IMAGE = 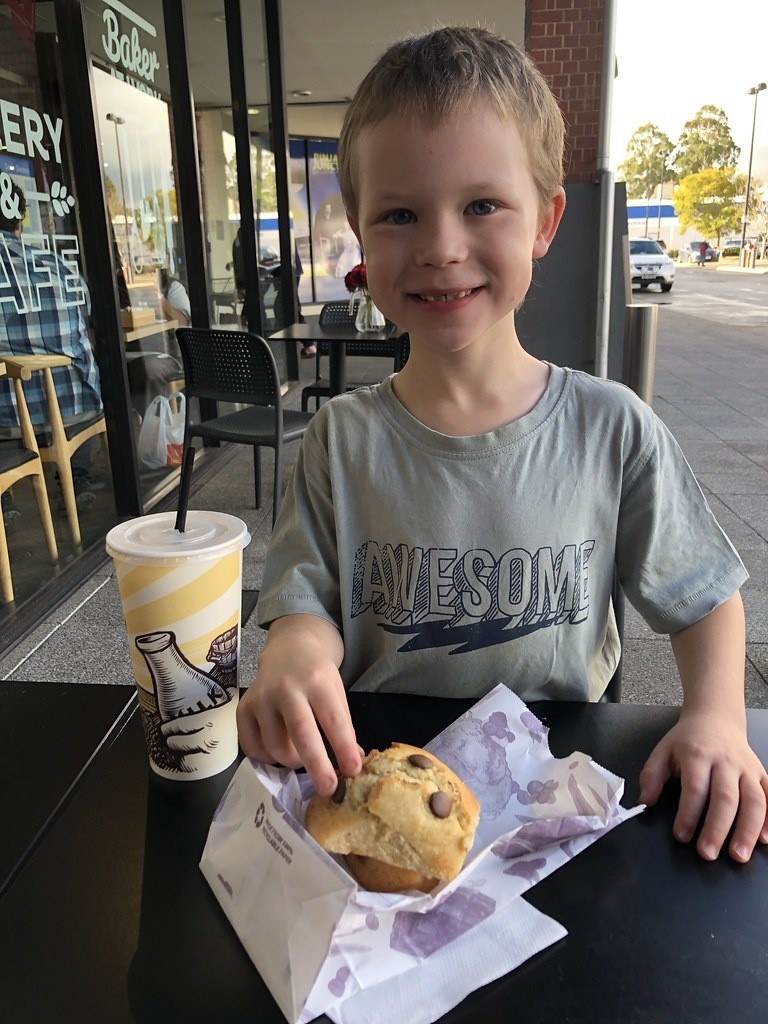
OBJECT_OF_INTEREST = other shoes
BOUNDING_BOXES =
[57,479,96,517]
[0,491,21,525]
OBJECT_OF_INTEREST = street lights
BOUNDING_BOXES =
[740,81,768,252]
[105,111,135,283]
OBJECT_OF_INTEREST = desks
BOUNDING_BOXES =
[87,319,180,342]
[0,679,139,887]
[269,323,395,398]
[0,690,768,1024]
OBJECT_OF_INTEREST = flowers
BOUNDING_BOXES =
[345,263,374,328]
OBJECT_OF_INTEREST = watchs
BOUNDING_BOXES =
[151,292,165,300]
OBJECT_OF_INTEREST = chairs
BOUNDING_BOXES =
[302,299,410,413]
[0,352,114,605]
[175,326,317,527]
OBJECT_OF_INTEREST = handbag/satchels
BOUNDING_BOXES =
[139,393,185,468]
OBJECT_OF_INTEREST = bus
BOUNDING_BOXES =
[230,210,296,271]
[112,213,179,274]
[724,236,757,249]
[627,197,702,253]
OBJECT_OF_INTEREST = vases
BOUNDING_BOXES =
[348,286,386,333]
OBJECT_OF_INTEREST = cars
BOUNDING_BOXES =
[655,240,666,250]
[678,240,716,263]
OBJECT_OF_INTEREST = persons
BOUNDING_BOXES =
[234,23,768,861]
[697,239,709,267]
[316,202,364,279]
[118,230,193,419]
[266,234,318,359]
[0,178,109,526]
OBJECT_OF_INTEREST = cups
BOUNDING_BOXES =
[106,509,253,782]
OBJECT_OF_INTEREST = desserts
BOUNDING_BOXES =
[304,742,480,892]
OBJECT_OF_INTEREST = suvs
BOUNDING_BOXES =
[630,236,675,293]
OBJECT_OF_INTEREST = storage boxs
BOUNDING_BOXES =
[120,309,156,327]
